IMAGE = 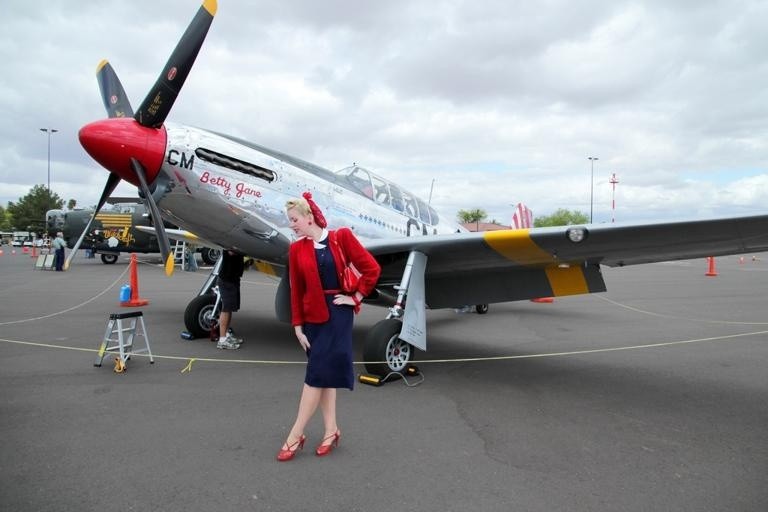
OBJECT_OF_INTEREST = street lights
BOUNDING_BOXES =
[588,156,599,224]
[39,128,58,199]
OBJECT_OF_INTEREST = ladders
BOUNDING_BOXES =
[94,311,154,370]
[173,226,186,271]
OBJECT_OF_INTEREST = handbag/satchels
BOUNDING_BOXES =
[334,228,363,293]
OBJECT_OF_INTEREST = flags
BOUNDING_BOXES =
[508,203,533,230]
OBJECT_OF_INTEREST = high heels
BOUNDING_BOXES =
[277,433,306,461]
[316,429,341,456]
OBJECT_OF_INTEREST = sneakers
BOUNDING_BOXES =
[217,335,244,350]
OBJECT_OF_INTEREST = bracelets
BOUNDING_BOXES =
[351,293,362,314]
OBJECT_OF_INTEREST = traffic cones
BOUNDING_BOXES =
[705,257,719,277]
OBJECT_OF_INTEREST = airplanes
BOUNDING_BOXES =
[63,0,768,381]
[41,195,255,271]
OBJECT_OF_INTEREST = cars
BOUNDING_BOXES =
[13,240,34,247]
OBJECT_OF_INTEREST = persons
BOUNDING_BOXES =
[216,249,245,351]
[20,236,26,247]
[274,195,383,462]
[52,232,68,271]
